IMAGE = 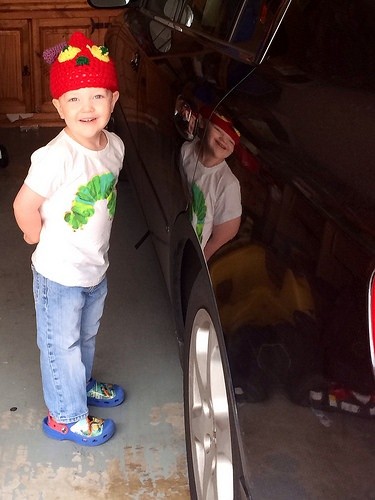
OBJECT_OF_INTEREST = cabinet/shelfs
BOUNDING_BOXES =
[0,0,125,128]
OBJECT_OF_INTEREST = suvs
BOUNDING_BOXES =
[89,0,375,500]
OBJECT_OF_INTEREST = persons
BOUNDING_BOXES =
[12,31,126,448]
[179,104,242,263]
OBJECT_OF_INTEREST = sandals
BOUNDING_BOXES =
[42,409,115,446]
[87,378,126,407]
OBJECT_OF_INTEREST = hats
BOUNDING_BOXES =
[200,104,241,146]
[49,31,120,99]
